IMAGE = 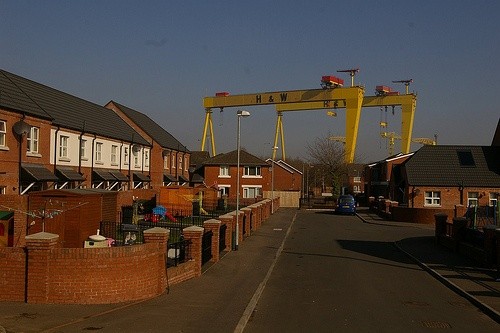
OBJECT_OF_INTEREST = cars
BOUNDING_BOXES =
[335,195,356,215]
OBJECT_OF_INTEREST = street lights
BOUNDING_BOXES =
[235,109,249,250]
